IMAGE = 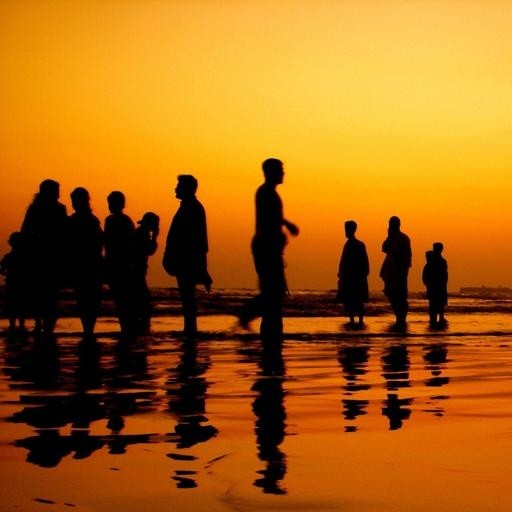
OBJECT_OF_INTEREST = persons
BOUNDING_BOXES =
[161,174,213,332]
[336,220,370,330]
[379,215,413,334]
[132,212,160,335]
[432,242,448,328]
[103,190,146,338]
[238,157,299,337]
[0,178,69,342]
[422,250,444,329]
[67,187,104,336]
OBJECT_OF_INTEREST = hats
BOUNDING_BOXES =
[137,212,159,224]
